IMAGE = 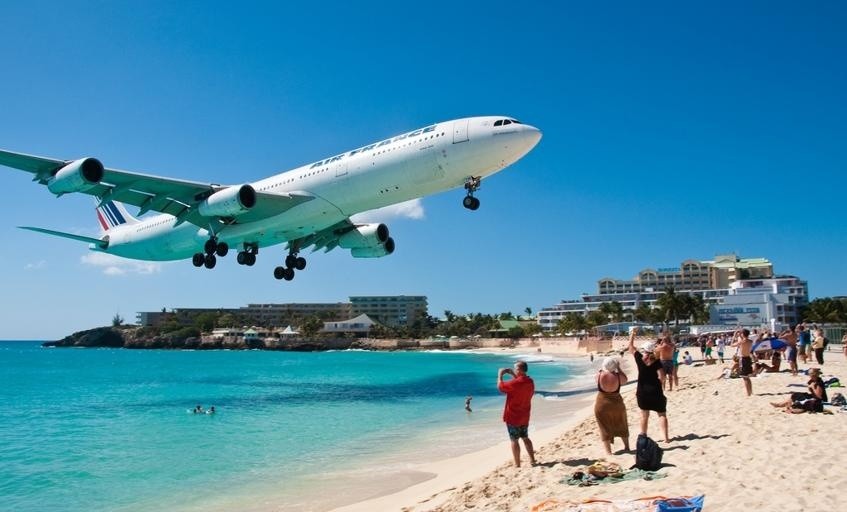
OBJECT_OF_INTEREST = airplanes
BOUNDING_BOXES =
[0,113,548,285]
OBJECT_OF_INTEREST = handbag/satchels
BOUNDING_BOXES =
[652,493,705,512]
[588,461,625,479]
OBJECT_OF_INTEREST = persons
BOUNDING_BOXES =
[653,321,846,397]
[197,406,205,413]
[593,355,630,457]
[627,322,672,445]
[193,409,204,414]
[768,367,827,414]
[464,396,473,413]
[495,361,537,469]
[206,406,215,414]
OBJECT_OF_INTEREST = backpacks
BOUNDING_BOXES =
[629,434,663,471]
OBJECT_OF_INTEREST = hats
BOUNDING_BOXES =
[640,341,656,353]
[602,357,619,374]
